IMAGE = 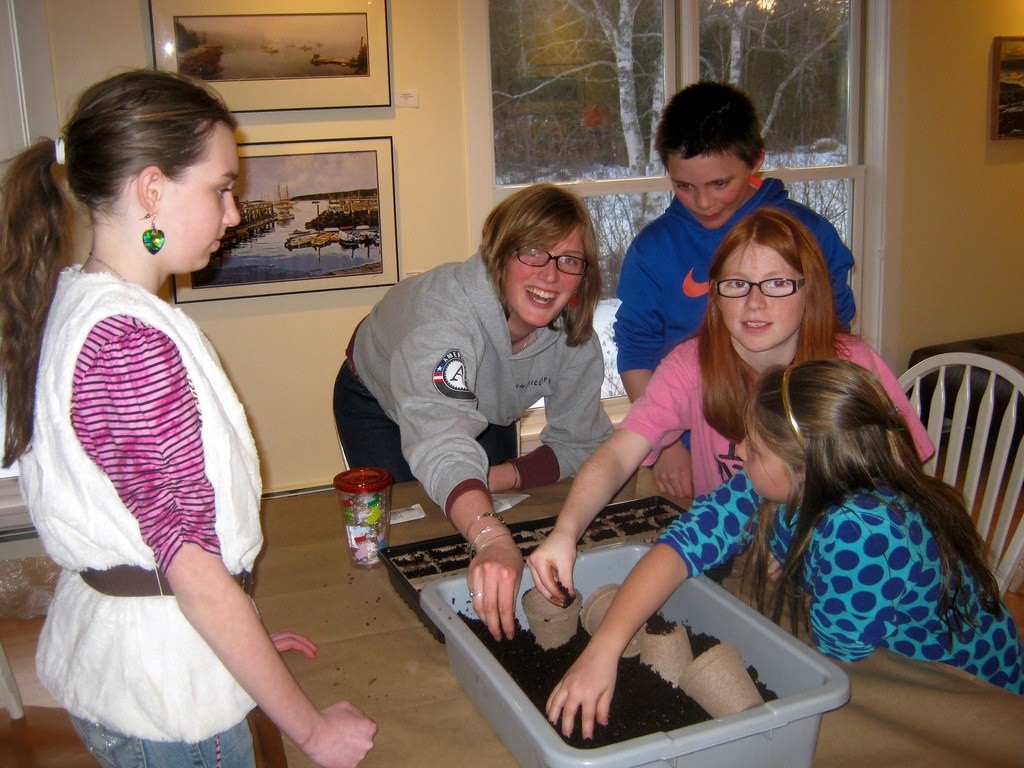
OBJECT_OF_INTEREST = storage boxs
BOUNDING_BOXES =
[378,495,734,644]
[419,540,854,768]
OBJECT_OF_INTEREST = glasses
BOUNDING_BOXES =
[515,246,588,275]
[714,278,805,298]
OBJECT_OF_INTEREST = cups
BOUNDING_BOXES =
[581,584,656,658]
[521,584,582,652]
[333,467,392,569]
[640,624,694,689]
[678,642,764,718]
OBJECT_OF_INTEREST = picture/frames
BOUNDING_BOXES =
[148,0,391,113]
[173,136,399,304]
[991,35,1024,140]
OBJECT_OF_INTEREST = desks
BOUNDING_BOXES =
[252,467,1024,768]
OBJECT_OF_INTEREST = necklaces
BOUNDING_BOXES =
[522,331,533,350]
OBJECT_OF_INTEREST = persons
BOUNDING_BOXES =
[333,183,615,641]
[612,80,856,499]
[544,357,1024,742]
[0,68,378,768]
[528,208,936,608]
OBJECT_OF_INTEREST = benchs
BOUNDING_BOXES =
[909,333,1024,445]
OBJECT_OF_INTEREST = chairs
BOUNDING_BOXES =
[898,352,1024,601]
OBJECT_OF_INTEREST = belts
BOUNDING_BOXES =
[79,564,252,597]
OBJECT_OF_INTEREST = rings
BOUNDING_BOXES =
[469,592,483,596]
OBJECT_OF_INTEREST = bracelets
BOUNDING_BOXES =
[466,513,511,560]
[506,461,517,489]
